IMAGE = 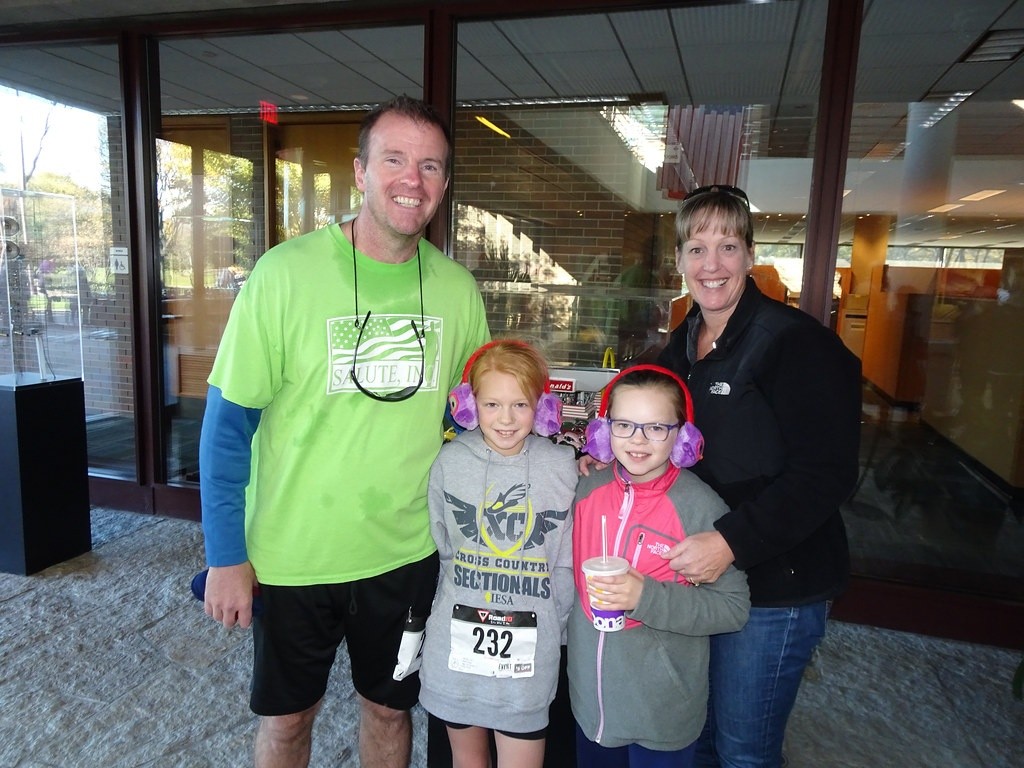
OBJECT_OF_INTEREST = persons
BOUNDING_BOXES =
[418,336,583,768]
[566,364,750,768]
[577,185,862,768]
[613,248,668,358]
[192,93,493,768]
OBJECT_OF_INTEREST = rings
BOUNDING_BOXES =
[688,577,700,587]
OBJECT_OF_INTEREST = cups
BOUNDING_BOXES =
[581,556,629,632]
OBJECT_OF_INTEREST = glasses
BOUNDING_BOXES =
[673,183,751,202]
[349,309,425,402]
[606,413,684,443]
